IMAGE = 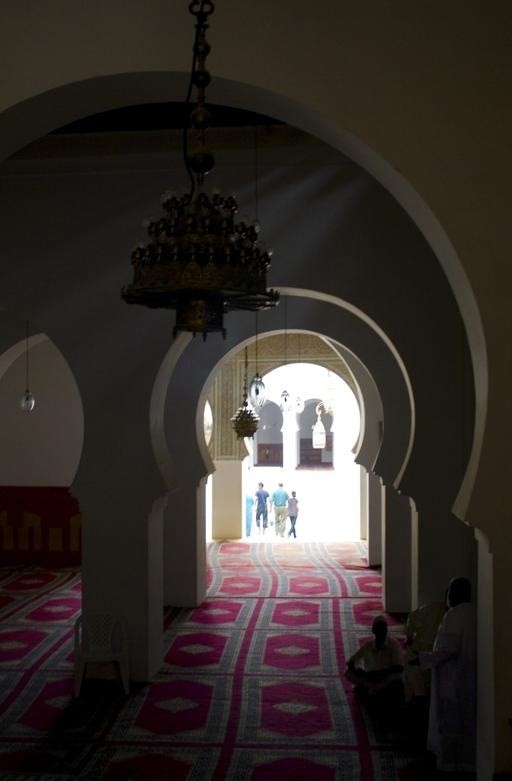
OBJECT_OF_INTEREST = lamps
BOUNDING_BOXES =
[247,312,269,409]
[311,401,331,450]
[21,321,37,415]
[117,0,282,347]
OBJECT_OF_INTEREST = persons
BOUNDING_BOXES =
[345,574,478,781]
[246,495,254,537]
[255,482,269,534]
[288,491,298,538]
[269,482,289,538]
